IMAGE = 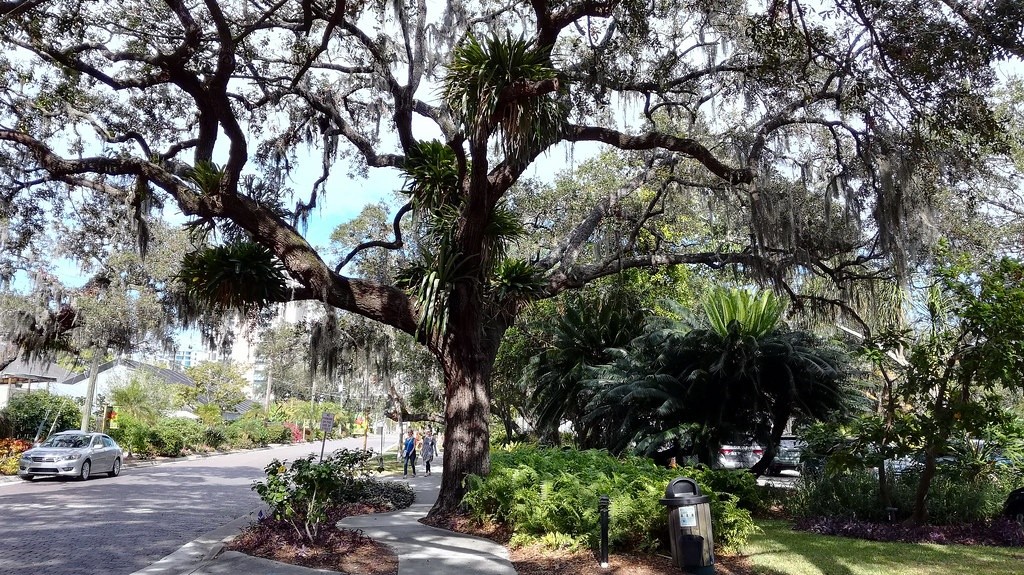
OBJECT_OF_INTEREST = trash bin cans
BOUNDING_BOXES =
[655,478,715,574]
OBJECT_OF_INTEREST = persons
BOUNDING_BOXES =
[403,428,438,479]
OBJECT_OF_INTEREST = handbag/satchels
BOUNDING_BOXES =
[401,450,408,458]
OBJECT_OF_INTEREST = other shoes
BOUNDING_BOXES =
[427,471,432,475]
[403,474,408,479]
[424,472,428,477]
[411,473,415,478]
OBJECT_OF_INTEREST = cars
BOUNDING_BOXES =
[718,432,807,479]
[16,432,123,482]
